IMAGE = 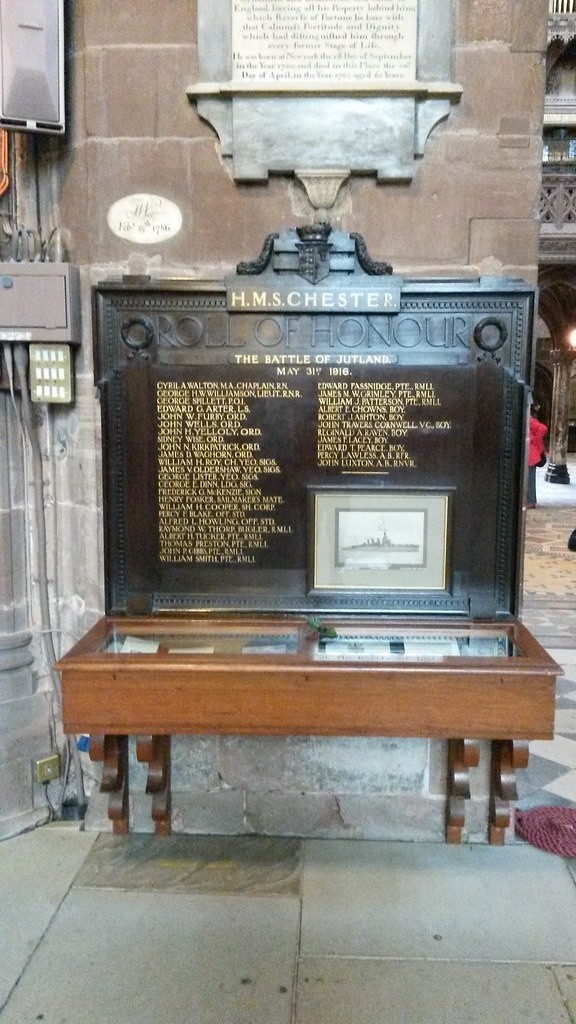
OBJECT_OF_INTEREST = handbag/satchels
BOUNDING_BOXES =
[536,451,546,467]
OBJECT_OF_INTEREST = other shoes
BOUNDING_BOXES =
[526,503,534,509]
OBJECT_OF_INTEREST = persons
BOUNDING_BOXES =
[525,404,548,508]
